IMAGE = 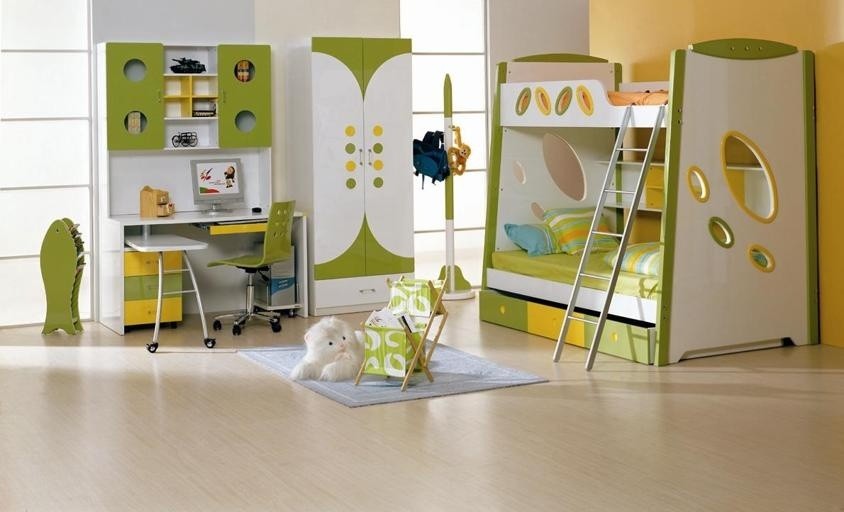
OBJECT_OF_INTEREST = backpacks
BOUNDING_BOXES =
[414,131,450,183]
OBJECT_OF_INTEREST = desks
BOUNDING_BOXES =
[125,233,217,354]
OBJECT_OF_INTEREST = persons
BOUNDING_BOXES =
[224,165,235,179]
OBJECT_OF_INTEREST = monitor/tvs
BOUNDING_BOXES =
[189,158,245,215]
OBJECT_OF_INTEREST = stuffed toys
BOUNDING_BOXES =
[446,126,472,175]
[290,316,368,383]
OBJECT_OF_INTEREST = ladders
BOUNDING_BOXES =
[551,105,667,372]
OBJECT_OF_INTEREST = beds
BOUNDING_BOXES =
[478,37,821,367]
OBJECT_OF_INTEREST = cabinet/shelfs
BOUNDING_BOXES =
[354,274,450,392]
[41,218,87,336]
[288,36,416,318]
[97,41,309,337]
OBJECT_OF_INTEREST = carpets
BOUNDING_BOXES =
[236,336,550,409]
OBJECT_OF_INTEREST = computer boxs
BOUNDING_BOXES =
[254,243,295,307]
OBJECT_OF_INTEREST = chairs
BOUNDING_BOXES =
[205,199,297,336]
